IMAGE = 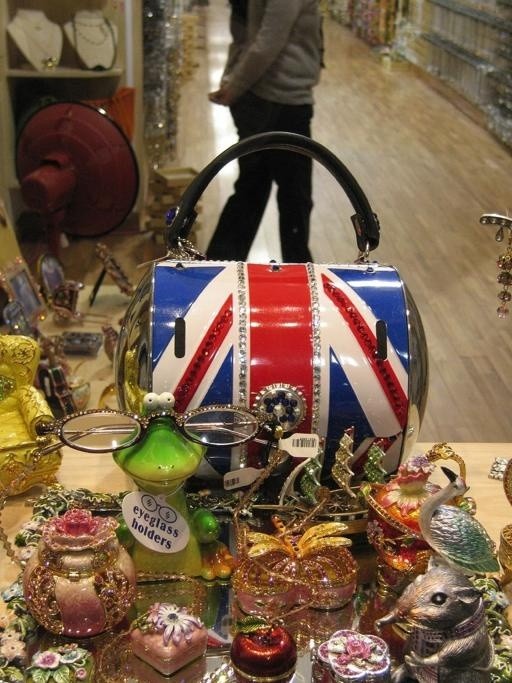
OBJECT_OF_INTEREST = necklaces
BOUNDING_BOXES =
[73,13,118,69]
[16,12,60,74]
[18,14,49,32]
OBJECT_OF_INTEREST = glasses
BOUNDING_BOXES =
[32,403,277,463]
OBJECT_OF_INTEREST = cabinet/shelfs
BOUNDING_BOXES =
[382,0,512,149]
[1,0,149,292]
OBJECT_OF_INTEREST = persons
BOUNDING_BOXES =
[205,0,327,263]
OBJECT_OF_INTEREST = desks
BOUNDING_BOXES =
[1,283,512,683]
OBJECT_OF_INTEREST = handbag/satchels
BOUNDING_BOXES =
[114,130,429,474]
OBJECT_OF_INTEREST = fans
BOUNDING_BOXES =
[14,100,140,265]
